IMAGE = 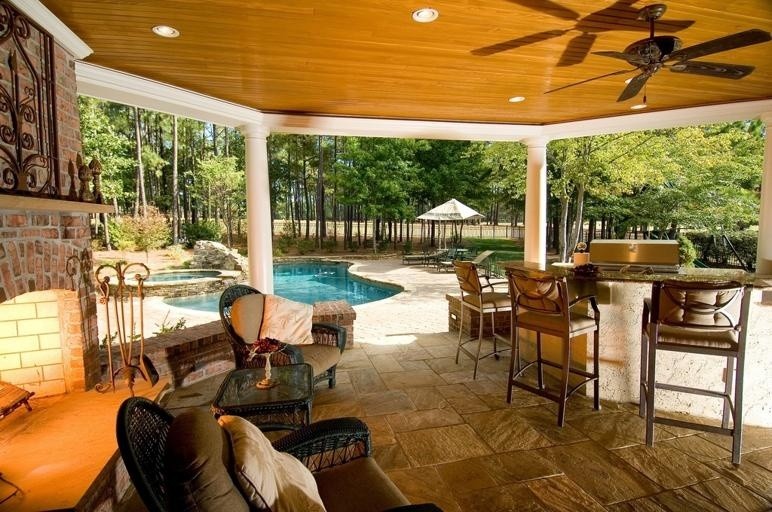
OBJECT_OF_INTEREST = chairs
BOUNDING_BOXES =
[405,245,498,278]
[501,262,606,426]
[115,394,440,511]
[638,278,753,468]
[452,257,514,383]
[217,281,345,395]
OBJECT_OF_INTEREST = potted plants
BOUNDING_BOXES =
[572,240,590,266]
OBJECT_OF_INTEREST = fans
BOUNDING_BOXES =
[543,2,770,101]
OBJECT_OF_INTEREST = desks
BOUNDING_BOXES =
[543,255,771,431]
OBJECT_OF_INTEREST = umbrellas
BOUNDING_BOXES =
[416,198,486,251]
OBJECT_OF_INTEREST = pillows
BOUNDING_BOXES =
[256,295,316,349]
[162,406,248,512]
[229,291,263,344]
[217,412,327,512]
